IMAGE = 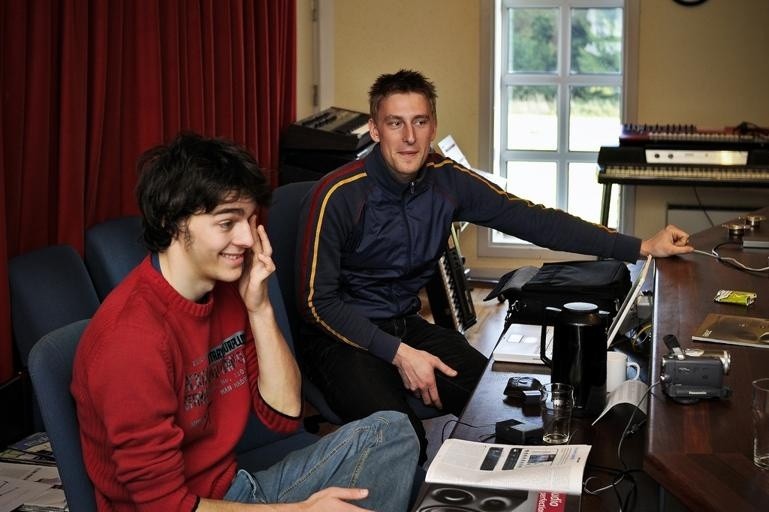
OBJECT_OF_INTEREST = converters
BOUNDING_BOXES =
[495,417,543,446]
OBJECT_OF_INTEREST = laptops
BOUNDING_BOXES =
[493,255,652,365]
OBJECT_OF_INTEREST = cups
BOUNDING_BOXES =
[539,382,575,444]
[751,378,769,472]
[607,352,640,394]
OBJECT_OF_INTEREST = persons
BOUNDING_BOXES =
[71,131,420,512]
[293,68,694,420]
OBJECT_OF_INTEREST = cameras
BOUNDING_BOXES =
[660,335,734,406]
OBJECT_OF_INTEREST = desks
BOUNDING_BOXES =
[411,216,769,512]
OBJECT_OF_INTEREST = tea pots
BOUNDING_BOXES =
[539,300,611,411]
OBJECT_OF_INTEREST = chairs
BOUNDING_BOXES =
[268,181,448,435]
[8,245,100,366]
[84,216,150,305]
[28,318,96,512]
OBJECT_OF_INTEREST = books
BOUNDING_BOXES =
[413,437,597,512]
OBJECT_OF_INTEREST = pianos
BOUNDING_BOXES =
[425,222,477,335]
[280,106,377,182]
[598,147,769,187]
[619,122,767,150]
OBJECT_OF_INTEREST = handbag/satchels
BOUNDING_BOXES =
[483,259,630,313]
